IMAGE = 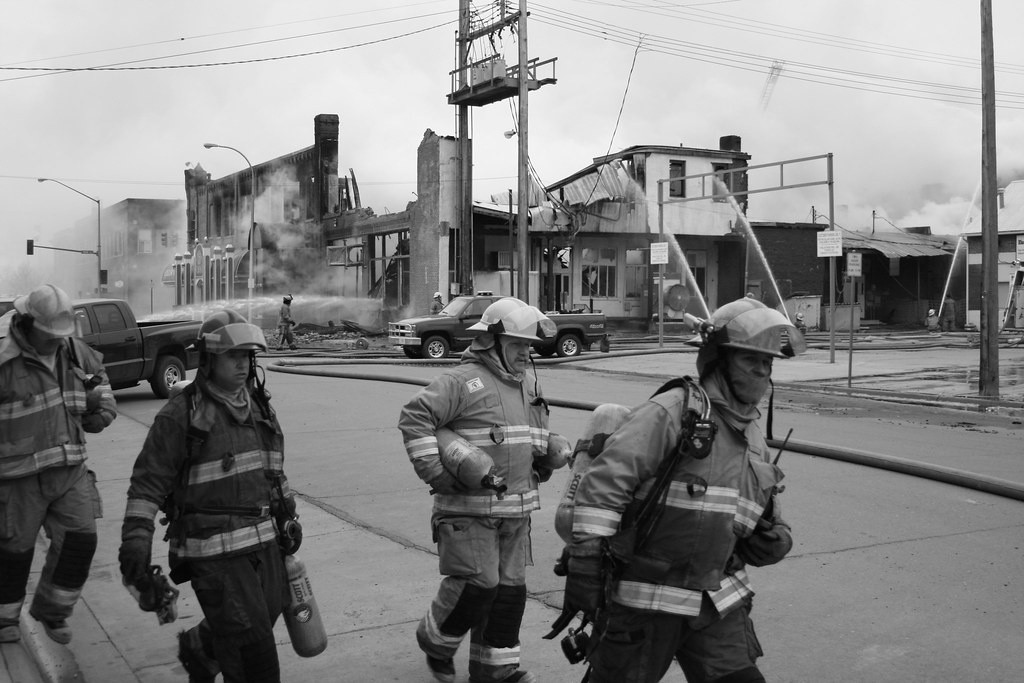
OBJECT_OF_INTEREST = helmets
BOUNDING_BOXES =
[183,310,264,353]
[13,284,76,336]
[465,295,543,341]
[683,297,790,359]
[283,293,293,300]
[433,291,442,300]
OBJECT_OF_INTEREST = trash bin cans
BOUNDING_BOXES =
[775,292,823,332]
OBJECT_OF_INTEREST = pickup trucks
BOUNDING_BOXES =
[388,290,611,360]
[68,298,202,399]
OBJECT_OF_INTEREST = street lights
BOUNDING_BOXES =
[204,142,255,323]
[35,178,104,299]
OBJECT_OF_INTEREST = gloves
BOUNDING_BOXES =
[532,455,553,482]
[119,541,152,580]
[291,321,296,326]
[563,556,601,615]
[274,496,302,546]
[81,414,108,433]
[748,533,793,567]
[429,471,466,495]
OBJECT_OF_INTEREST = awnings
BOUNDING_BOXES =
[247,221,303,249]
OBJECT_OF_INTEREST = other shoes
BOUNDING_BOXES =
[178,632,216,683]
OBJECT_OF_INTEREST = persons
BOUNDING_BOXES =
[567,298,807,683]
[925,309,941,331]
[794,312,806,334]
[942,291,958,332]
[277,294,300,350]
[430,292,444,314]
[118,309,303,683]
[397,298,572,683]
[0,283,119,644]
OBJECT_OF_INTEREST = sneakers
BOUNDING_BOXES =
[0,620,20,643]
[426,654,456,683]
[469,670,536,683]
[29,607,72,643]
